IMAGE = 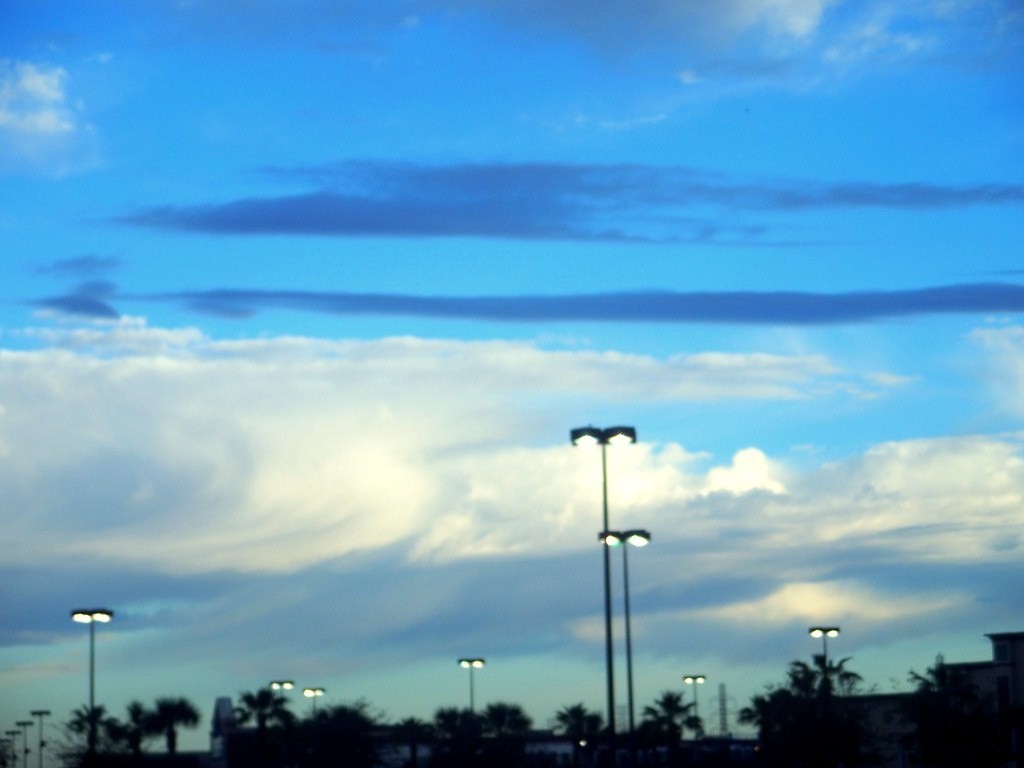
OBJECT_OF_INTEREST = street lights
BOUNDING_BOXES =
[70,607,115,710]
[268,680,295,710]
[596,529,654,768]
[567,425,640,768]
[31,709,52,768]
[806,624,841,680]
[303,687,327,722]
[16,720,35,768]
[5,730,22,768]
[681,674,707,743]
[456,656,486,712]
